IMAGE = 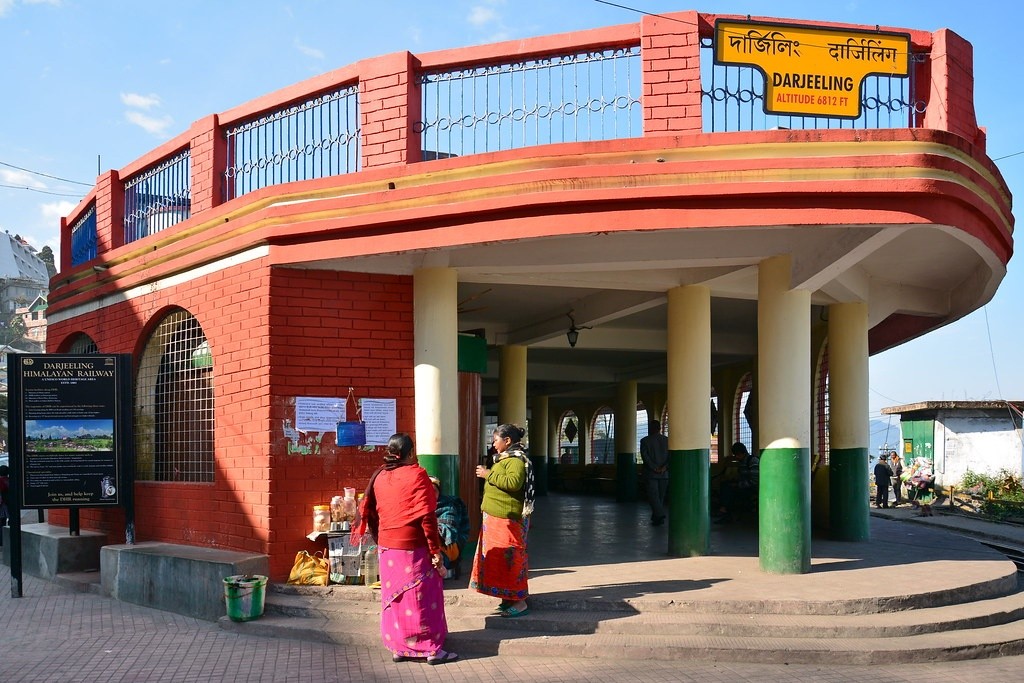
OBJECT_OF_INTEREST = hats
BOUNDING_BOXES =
[428,475,442,491]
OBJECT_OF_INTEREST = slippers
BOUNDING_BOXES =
[393,657,415,662]
[501,606,529,618]
[490,604,509,615]
[427,651,459,665]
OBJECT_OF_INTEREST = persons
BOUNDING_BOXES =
[561,448,574,465]
[874,455,894,509]
[640,420,671,526]
[0,465,9,546]
[711,442,760,524]
[349,432,459,665]
[428,475,470,570]
[466,424,535,619]
[889,451,907,507]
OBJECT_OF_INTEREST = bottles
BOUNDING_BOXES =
[365,546,378,585]
[313,486,365,533]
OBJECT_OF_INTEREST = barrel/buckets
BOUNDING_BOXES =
[223,575,268,622]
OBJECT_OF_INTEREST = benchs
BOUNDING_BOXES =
[588,477,615,493]
